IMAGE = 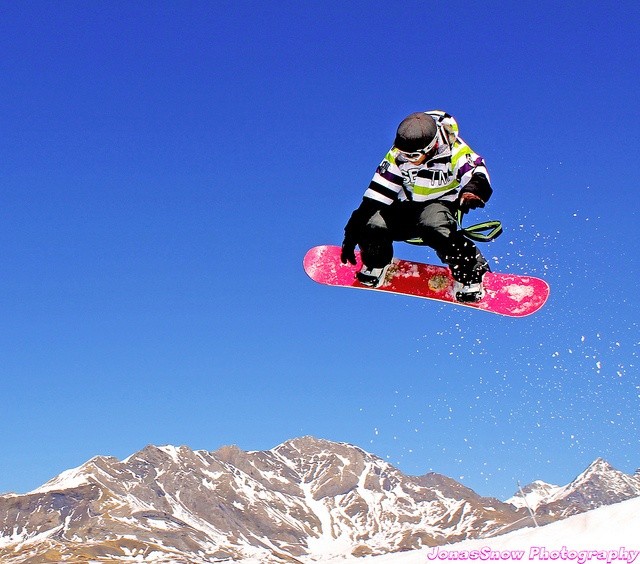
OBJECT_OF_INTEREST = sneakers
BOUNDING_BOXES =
[356,258,393,288]
[452,269,485,302]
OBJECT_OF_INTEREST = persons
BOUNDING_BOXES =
[340,111,494,303]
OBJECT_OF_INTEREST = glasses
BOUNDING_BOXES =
[390,146,424,162]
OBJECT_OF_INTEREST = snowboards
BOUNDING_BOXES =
[303,244,550,318]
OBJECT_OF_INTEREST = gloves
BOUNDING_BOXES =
[457,192,486,213]
[341,229,360,265]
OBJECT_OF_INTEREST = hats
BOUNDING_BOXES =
[395,113,438,153]
